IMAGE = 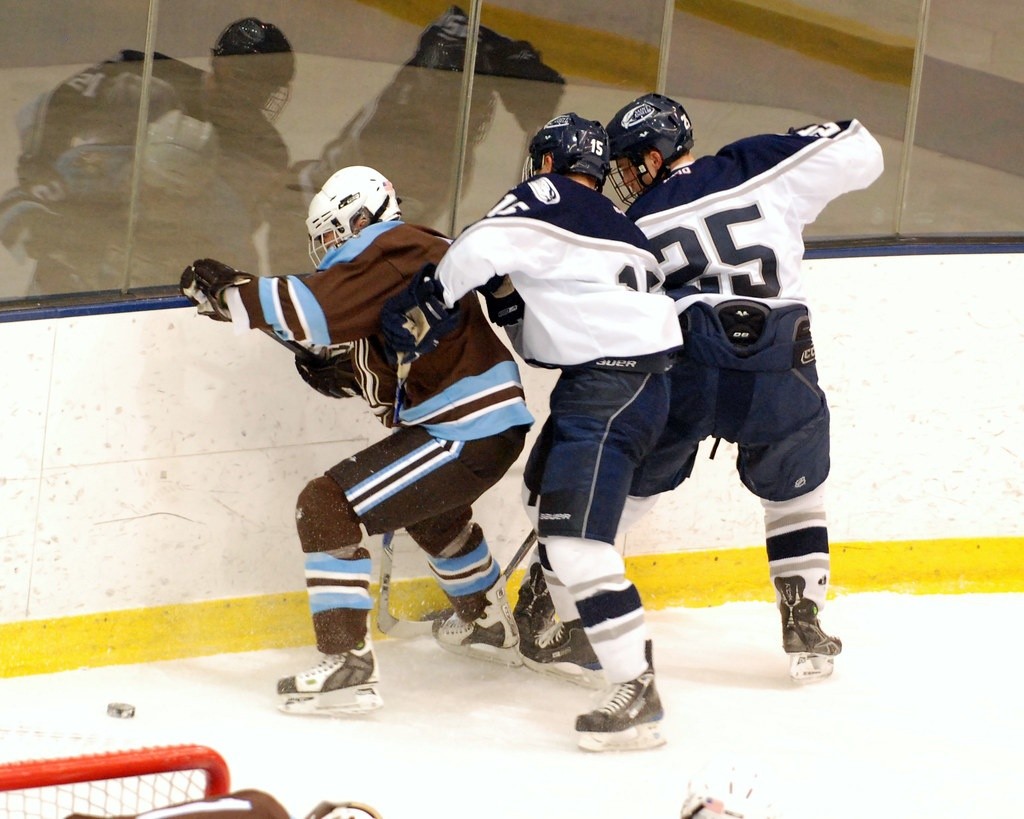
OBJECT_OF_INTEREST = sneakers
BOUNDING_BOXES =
[513,563,556,641]
[518,620,606,685]
[432,577,525,666]
[573,638,668,752]
[776,574,839,686]
[274,638,380,715]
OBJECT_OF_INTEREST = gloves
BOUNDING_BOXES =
[180,257,253,322]
[379,262,459,366]
[294,341,361,398]
[475,273,524,327]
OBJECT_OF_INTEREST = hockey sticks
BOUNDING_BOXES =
[432,529,536,639]
[255,319,320,362]
[376,352,446,637]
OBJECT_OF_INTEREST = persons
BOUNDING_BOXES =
[378,112,684,751]
[178,166,535,716]
[513,93,884,683]
[0,5,566,292]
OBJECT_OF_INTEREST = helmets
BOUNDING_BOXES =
[211,16,295,128]
[143,110,216,199]
[305,164,400,272]
[519,111,610,191]
[604,93,694,206]
[422,38,492,112]
